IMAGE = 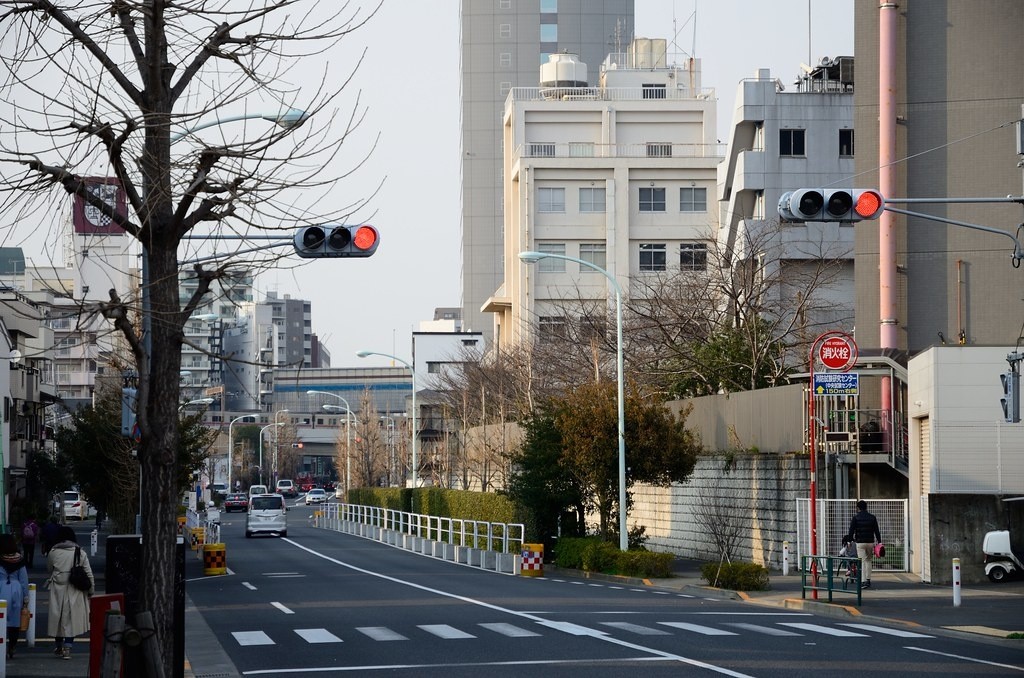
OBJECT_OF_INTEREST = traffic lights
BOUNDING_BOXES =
[999,370,1021,423]
[789,188,885,220]
[292,444,298,448]
[295,223,379,255]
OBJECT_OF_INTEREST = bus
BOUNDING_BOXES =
[225,493,249,513]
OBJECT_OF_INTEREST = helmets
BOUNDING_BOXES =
[875,544,885,558]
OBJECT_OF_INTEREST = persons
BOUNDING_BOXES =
[840,501,881,589]
[45,516,58,540]
[0,534,29,658]
[21,513,39,569]
[96,508,103,530]
[45,527,95,659]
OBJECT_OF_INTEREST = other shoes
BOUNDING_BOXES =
[62,648,72,659]
[846,570,850,575]
[7,646,17,654]
[54,647,63,656]
[861,579,871,589]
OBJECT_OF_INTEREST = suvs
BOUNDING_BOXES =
[276,479,299,498]
[206,482,227,500]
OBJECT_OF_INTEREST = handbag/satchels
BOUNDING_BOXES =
[19,602,30,632]
[69,547,92,592]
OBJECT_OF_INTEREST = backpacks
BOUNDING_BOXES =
[23,523,35,537]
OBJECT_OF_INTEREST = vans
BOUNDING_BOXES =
[248,484,268,500]
[245,493,291,538]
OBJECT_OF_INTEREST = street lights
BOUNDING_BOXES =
[275,409,290,486]
[354,350,417,490]
[381,416,395,469]
[142,105,313,380]
[229,413,260,493]
[517,250,629,555]
[306,390,351,490]
[322,404,356,441]
[259,422,286,485]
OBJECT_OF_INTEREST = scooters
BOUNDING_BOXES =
[983,529,1024,584]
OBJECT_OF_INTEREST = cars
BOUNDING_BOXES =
[305,488,328,505]
[55,491,89,521]
[302,482,334,493]
